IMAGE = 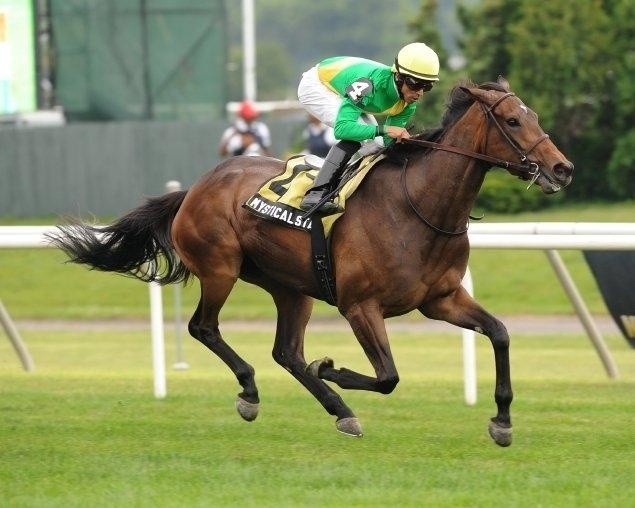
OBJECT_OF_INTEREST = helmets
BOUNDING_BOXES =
[389,41,442,82]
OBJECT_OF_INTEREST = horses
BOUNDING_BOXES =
[41,74,576,449]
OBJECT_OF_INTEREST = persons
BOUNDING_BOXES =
[301,112,342,159]
[219,100,271,159]
[298,42,440,213]
[237,129,266,156]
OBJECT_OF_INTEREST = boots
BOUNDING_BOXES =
[299,144,354,215]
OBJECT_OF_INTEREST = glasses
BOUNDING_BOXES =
[401,74,434,92]
